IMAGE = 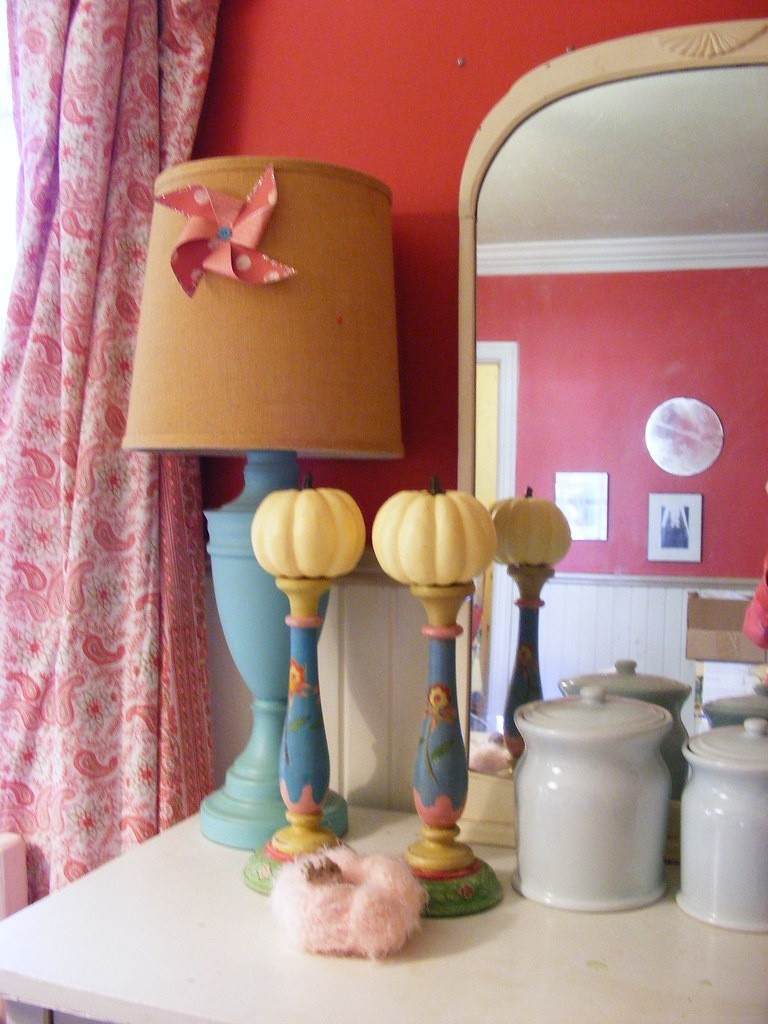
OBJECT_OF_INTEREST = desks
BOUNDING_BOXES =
[0,806,768,1024]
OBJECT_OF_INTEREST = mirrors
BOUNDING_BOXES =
[644,396,723,477]
[452,17,768,851]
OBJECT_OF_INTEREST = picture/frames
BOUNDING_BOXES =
[647,492,702,563]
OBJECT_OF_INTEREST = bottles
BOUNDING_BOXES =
[672,718,768,935]
[701,682,768,730]
[513,684,675,912]
[556,659,696,801]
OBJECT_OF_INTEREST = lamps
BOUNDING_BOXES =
[124,153,408,859]
